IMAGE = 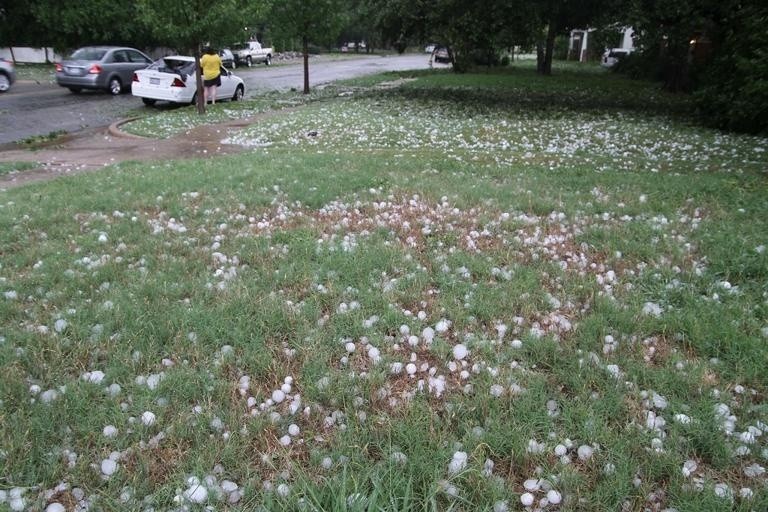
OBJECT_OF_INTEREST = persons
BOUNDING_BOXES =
[199,47,222,105]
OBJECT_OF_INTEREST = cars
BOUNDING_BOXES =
[217,47,237,69]
[435,47,452,65]
[131,55,246,106]
[0,57,17,92]
[56,45,155,95]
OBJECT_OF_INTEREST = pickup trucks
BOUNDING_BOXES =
[231,41,275,67]
